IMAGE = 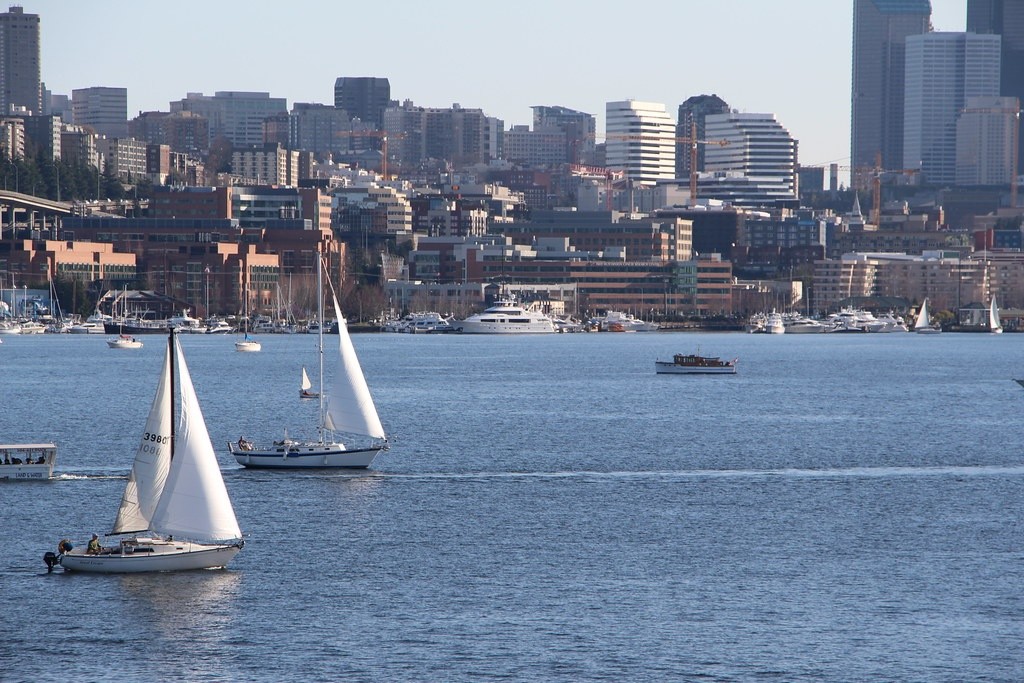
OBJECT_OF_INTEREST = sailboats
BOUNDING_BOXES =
[225,253,390,471]
[44,325,247,576]
[234,282,262,352]
[987,293,1006,335]
[298,366,320,399]
[106,296,144,349]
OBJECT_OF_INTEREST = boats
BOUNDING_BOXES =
[0,444,57,482]
[553,308,662,336]
[742,262,945,335]
[447,246,558,335]
[0,274,332,350]
[652,351,739,375]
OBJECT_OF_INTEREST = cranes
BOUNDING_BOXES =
[333,128,406,181]
[570,163,627,210]
[587,114,732,207]
[960,96,1024,210]
[792,148,920,231]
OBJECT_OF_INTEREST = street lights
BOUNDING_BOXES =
[88,163,98,201]
[44,164,60,203]
[10,162,19,193]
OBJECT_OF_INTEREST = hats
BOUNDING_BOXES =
[93,534,98,537]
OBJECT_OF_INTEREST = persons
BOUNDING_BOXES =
[238,436,249,451]
[87,533,106,555]
[5,457,44,465]
[303,390,308,395]
